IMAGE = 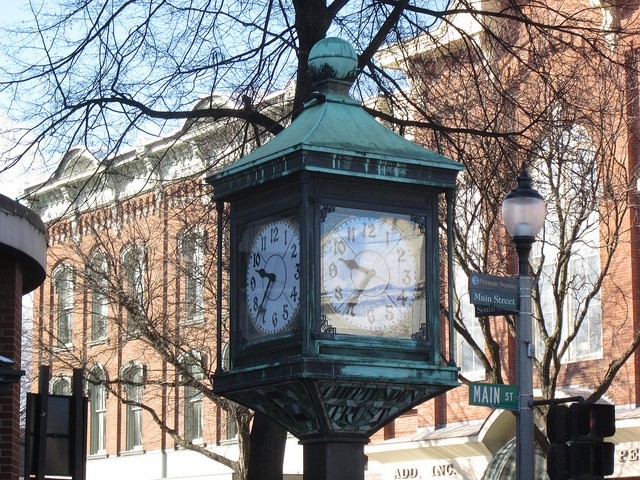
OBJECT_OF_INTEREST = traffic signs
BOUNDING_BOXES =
[468,272,519,296]
[468,381,519,410]
[474,305,519,317]
[470,288,520,313]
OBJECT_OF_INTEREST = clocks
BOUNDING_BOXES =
[314,196,433,349]
[231,197,304,353]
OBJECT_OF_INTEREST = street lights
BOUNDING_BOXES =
[502,159,546,480]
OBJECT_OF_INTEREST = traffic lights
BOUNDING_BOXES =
[570,401,616,479]
